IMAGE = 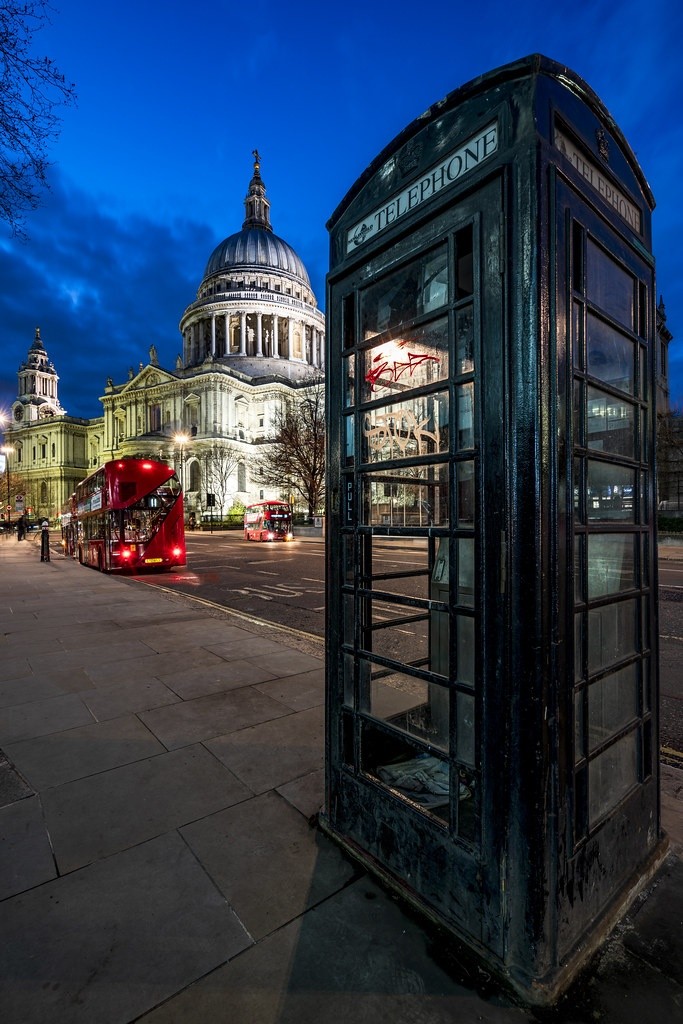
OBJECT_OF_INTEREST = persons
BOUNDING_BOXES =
[283,523,288,531]
[65,515,84,560]
[41,517,48,526]
[27,519,32,532]
[189,517,196,531]
[142,529,150,541]
[17,517,26,541]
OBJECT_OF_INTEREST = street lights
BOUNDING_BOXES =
[174,434,187,488]
[0,445,15,524]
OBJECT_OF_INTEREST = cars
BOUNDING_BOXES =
[0,522,40,533]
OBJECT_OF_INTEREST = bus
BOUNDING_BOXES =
[61,453,188,574]
[243,500,294,542]
